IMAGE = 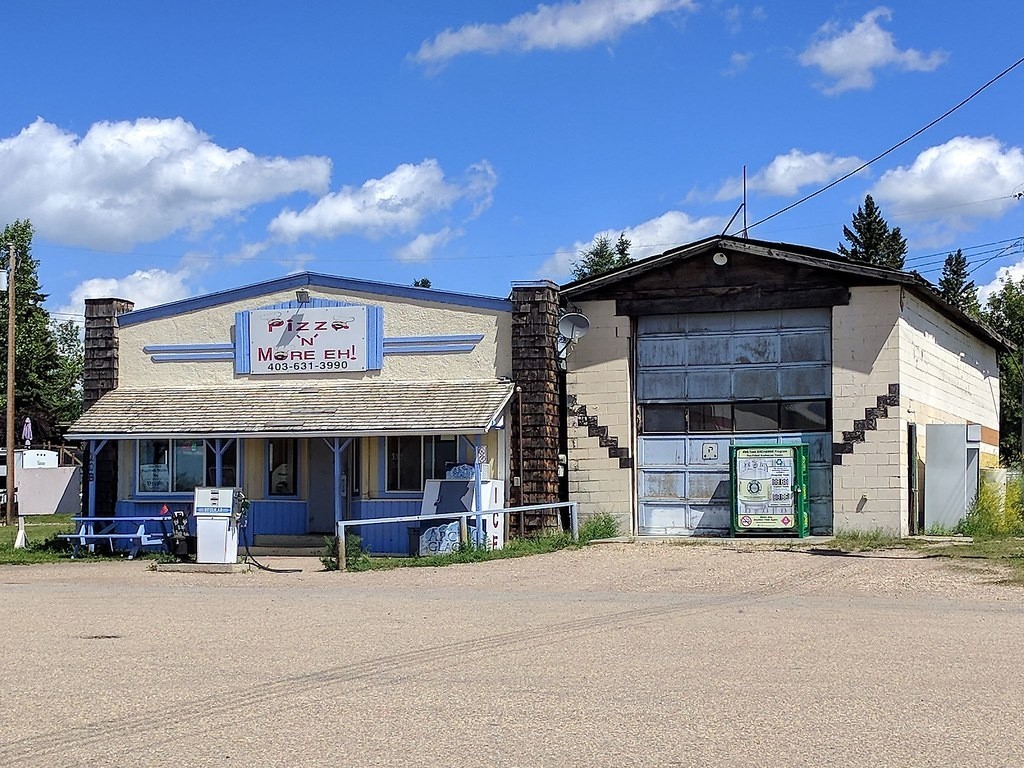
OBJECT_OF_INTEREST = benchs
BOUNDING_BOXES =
[56,534,152,539]
[151,533,173,537]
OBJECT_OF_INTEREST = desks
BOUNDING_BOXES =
[69,517,171,560]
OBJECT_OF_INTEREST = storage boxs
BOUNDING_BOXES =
[446,463,491,479]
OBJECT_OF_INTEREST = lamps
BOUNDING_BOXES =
[712,249,729,266]
[296,288,310,304]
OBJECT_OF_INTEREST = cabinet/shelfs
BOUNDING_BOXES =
[728,443,811,539]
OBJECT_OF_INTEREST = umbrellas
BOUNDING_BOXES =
[22,417,32,448]
